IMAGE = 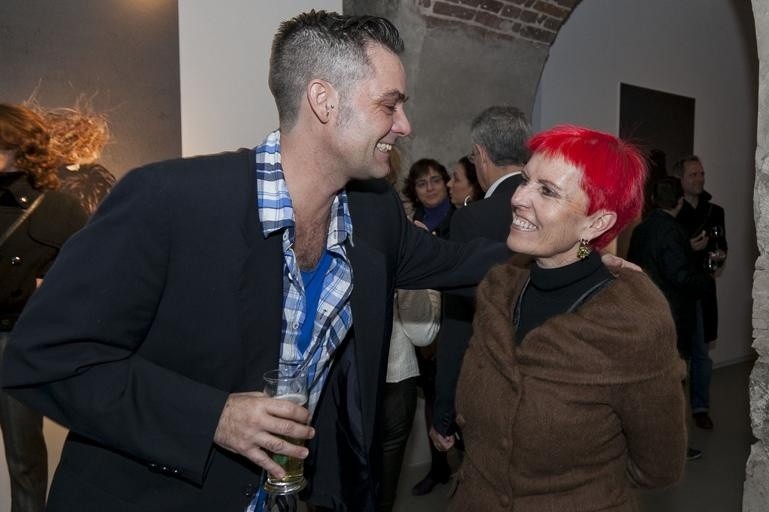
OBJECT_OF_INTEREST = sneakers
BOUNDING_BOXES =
[685,447,703,461]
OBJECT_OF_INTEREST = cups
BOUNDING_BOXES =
[263,368,307,496]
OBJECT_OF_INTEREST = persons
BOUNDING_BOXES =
[0,8,643,512]
[0,101,89,512]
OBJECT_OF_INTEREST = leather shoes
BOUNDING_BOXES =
[413,464,452,495]
[692,412,713,429]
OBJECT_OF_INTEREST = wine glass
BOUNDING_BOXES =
[703,258,718,281]
[711,225,724,256]
